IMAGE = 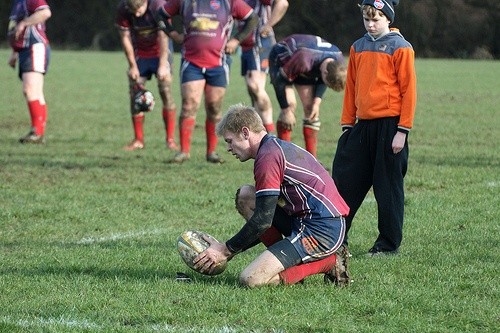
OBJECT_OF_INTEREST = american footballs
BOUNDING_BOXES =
[177,231,227,275]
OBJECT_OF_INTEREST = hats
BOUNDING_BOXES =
[133,82,155,111]
[361,0,399,23]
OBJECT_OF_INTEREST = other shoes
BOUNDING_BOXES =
[322,247,350,287]
[207,150,226,163]
[367,246,399,253]
[20,131,44,144]
[173,152,191,162]
[122,139,144,150]
[167,140,178,151]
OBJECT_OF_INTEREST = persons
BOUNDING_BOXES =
[268,34,348,159]
[332,0,417,253]
[235,0,290,134]
[114,0,179,151]
[192,102,350,288]
[8,0,51,144]
[154,0,259,164]
